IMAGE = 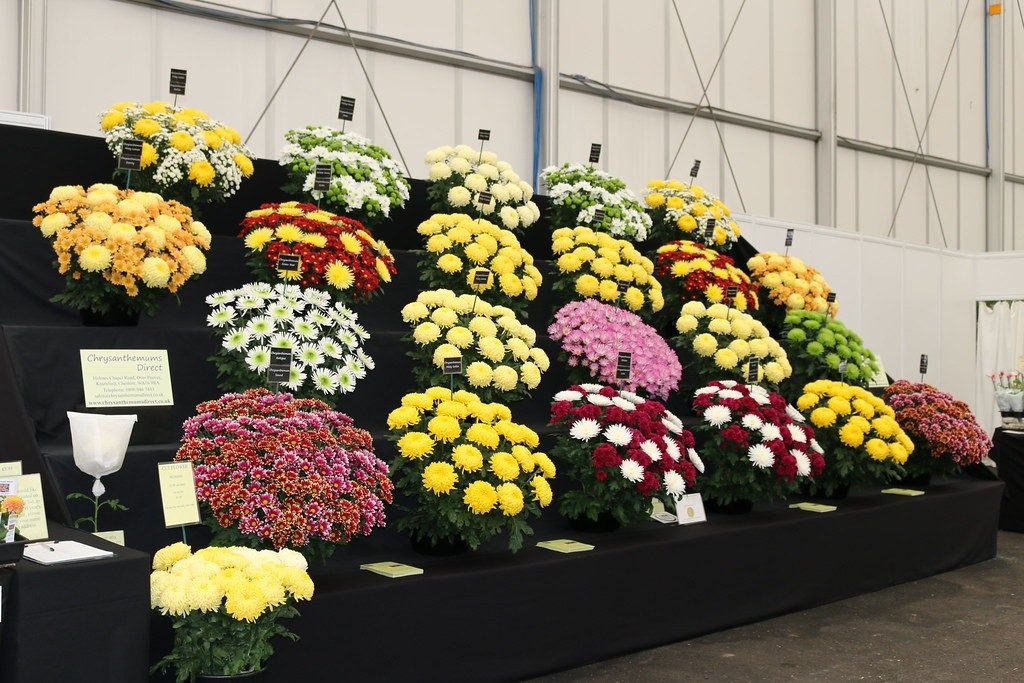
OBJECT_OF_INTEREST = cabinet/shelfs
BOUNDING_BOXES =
[0,124,1002,683]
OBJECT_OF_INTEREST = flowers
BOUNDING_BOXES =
[30,98,995,550]
[147,541,317,682]
[990,371,1024,410]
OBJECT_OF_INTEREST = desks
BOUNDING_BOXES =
[991,427,1024,534]
[7,522,149,683]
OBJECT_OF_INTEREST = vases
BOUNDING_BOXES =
[565,508,621,532]
[706,495,753,515]
[886,473,925,488]
[791,478,852,498]
[0,496,25,543]
[409,528,468,558]
[1001,411,1024,419]
[193,659,271,682]
[0,532,29,563]
[79,303,140,328]
